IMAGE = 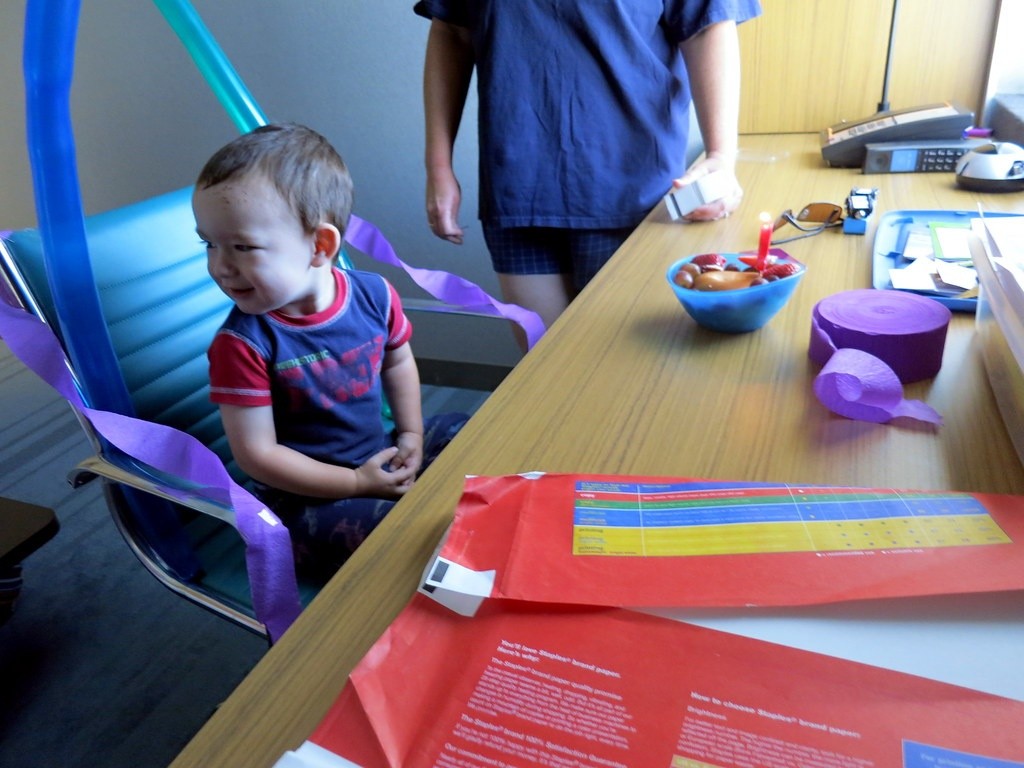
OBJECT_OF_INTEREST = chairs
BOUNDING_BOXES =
[0,180,548,640]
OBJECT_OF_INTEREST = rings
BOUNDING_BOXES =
[720,210,730,219]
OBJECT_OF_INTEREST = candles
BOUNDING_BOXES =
[755,212,773,270]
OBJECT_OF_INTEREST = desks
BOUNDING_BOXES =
[162,134,1024,768]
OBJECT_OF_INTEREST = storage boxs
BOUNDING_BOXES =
[967,216,1024,470]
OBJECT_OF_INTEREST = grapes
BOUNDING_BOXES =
[675,263,702,289]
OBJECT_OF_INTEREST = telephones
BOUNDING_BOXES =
[861,138,994,174]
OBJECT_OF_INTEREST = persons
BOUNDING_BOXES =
[189,121,478,585]
[411,1,763,351]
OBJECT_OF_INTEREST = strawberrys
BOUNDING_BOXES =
[690,253,726,272]
[762,262,800,278]
[738,255,778,268]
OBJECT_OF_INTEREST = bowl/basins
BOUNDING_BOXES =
[666,248,806,333]
